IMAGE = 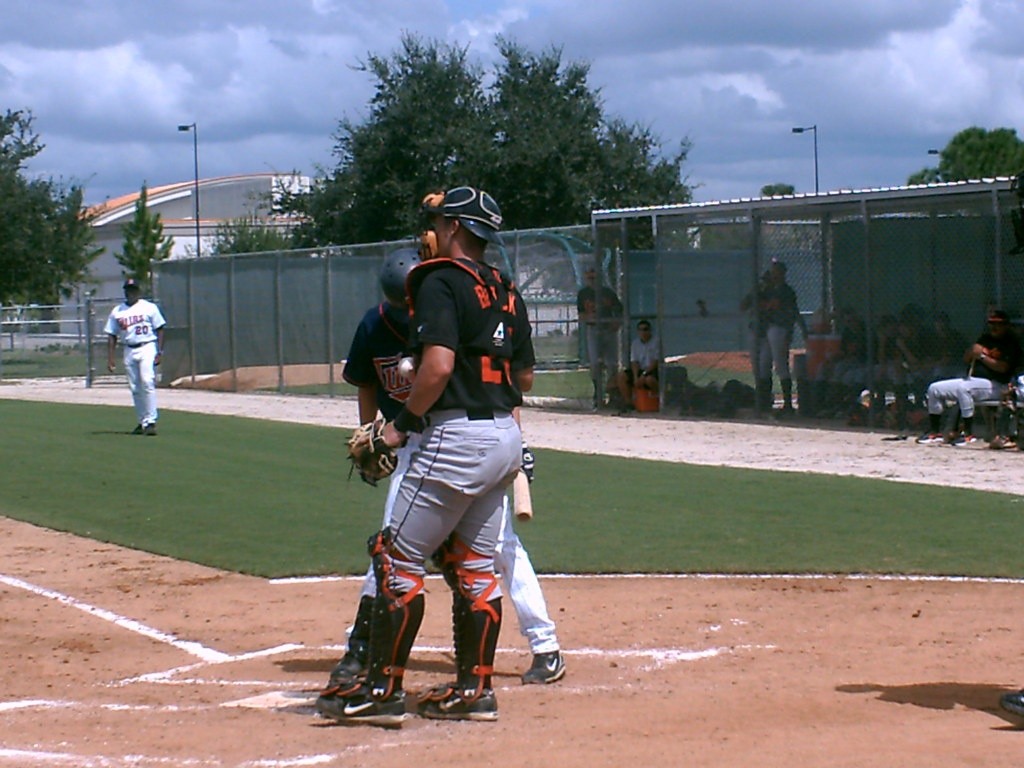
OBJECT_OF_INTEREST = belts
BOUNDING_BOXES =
[424,408,494,425]
[128,342,148,348]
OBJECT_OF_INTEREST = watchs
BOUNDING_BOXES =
[642,370,648,376]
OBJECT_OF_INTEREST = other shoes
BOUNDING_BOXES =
[621,405,634,412]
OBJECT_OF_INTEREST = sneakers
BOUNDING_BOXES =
[955,433,977,445]
[318,686,408,724]
[133,424,143,434]
[145,423,157,435]
[916,433,945,443]
[990,435,1016,449]
[417,681,498,719]
[328,672,367,687]
[521,649,565,683]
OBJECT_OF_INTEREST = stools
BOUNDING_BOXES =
[633,388,658,411]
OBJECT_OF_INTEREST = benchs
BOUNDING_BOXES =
[946,401,1024,439]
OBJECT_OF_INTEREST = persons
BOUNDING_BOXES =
[103,277,165,437]
[810,303,1024,451]
[741,259,812,421]
[615,320,662,409]
[316,186,570,728]
[576,259,625,409]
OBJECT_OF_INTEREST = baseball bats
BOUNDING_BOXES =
[952,356,977,431]
[512,465,533,523]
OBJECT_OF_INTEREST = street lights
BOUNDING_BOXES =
[791,124,819,194]
[176,122,201,256]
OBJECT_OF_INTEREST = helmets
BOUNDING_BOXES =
[382,249,424,301]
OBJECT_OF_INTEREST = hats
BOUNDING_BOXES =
[123,279,139,290]
[421,187,503,242]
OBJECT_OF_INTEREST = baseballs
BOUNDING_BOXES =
[398,357,414,377]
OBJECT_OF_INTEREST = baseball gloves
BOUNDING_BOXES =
[348,419,401,483]
